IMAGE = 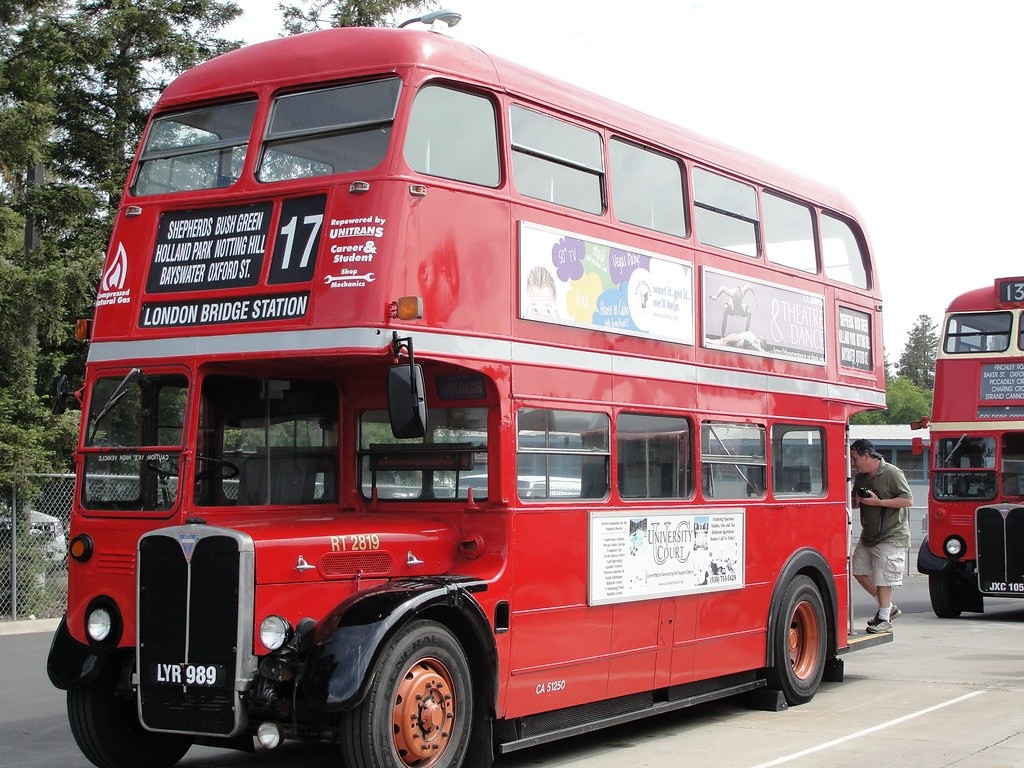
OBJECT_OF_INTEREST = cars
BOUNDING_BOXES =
[0,505,69,567]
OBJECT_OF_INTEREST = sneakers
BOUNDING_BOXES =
[866,604,902,625]
[869,621,894,633]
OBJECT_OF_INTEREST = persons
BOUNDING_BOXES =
[849,439,913,634]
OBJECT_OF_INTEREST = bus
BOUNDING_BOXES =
[913,273,1024,619]
[48,27,901,768]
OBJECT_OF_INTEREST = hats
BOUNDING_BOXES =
[848,437,884,459]
